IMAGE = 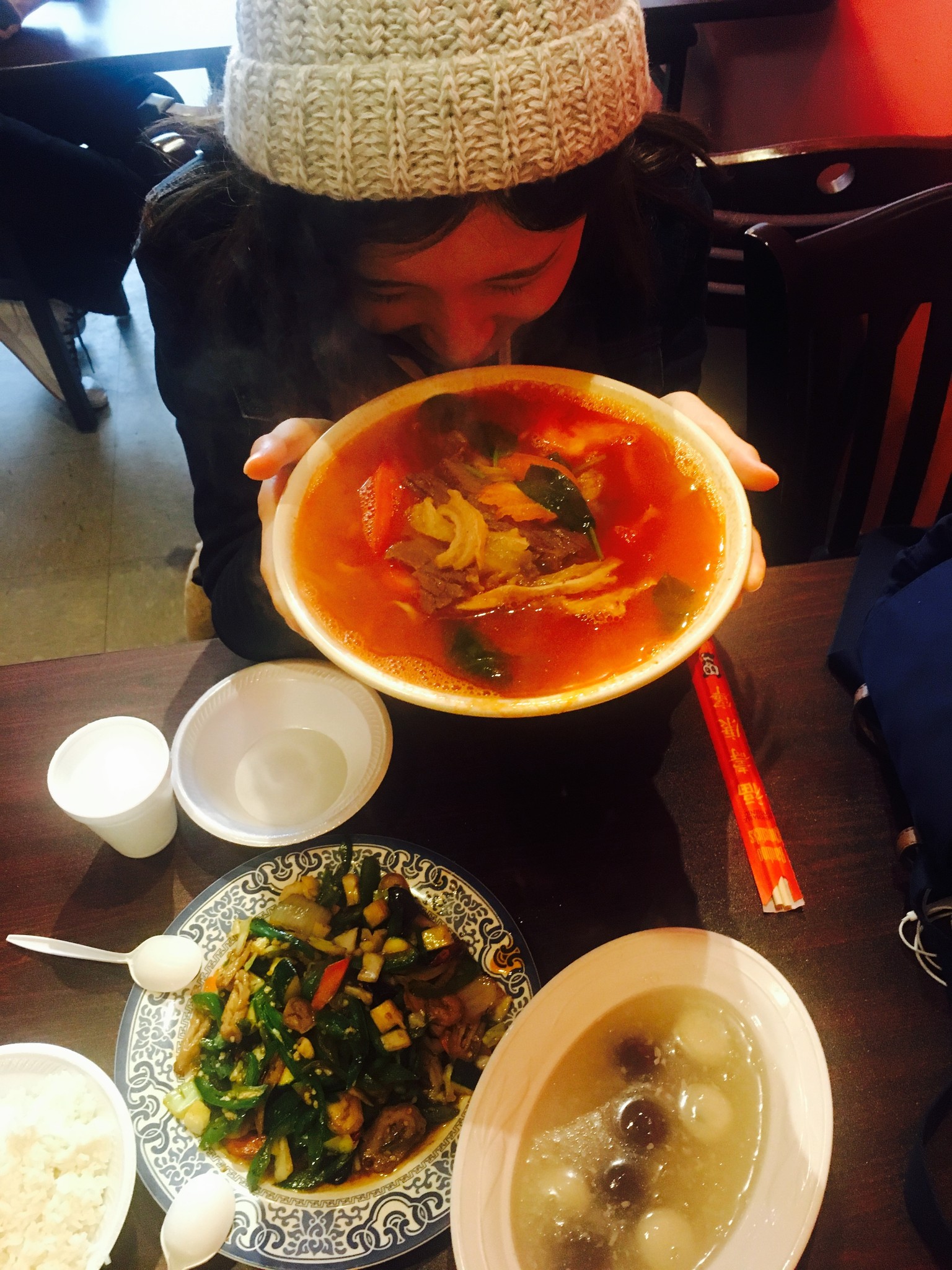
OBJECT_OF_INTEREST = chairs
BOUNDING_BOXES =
[685,131,952,569]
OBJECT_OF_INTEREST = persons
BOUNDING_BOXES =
[0,1,188,410]
[133,1,782,659]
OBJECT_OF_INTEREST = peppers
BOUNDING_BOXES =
[185,839,479,1193]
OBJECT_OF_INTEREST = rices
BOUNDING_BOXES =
[0,1066,114,1270]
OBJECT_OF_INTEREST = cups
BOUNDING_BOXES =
[48,714,178,858]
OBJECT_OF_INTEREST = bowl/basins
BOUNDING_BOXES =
[170,657,394,848]
[270,365,753,719]
[1,1043,137,1269]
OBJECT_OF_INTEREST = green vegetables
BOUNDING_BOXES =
[416,392,605,680]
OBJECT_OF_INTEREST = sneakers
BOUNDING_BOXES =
[0,296,109,408]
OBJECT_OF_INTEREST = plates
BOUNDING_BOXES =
[113,832,543,1270]
[450,927,833,1270]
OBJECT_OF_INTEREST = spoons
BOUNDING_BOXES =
[160,1175,236,1270]
[5,933,205,992]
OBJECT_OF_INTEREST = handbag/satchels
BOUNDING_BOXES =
[832,512,952,928]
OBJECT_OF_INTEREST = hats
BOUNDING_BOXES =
[220,1,650,201]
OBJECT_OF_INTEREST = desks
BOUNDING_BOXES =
[0,560,952,1269]
